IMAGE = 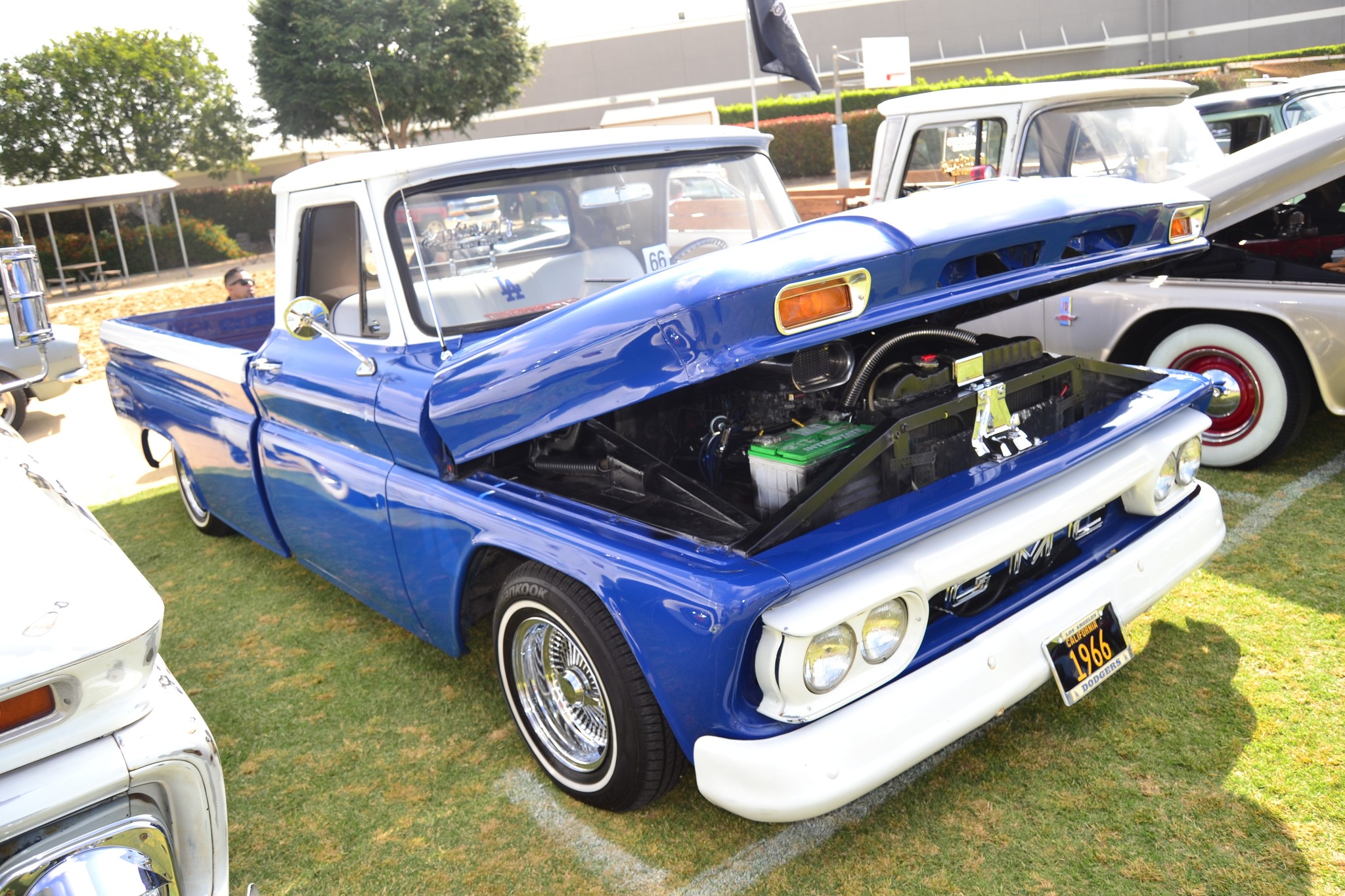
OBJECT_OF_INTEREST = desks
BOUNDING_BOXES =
[55,262,107,291]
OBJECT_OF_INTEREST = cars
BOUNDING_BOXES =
[0,322,87,432]
[0,419,235,896]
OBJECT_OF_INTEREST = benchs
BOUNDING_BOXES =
[91,269,124,286]
[329,245,645,339]
[46,277,76,296]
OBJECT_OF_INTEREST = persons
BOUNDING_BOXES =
[224,268,255,301]
[410,220,449,266]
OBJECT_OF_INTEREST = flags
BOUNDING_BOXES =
[748,0,822,96]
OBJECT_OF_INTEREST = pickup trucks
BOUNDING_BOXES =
[98,122,1231,824]
[670,74,1345,470]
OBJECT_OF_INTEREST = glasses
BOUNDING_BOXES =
[230,277,255,285]
